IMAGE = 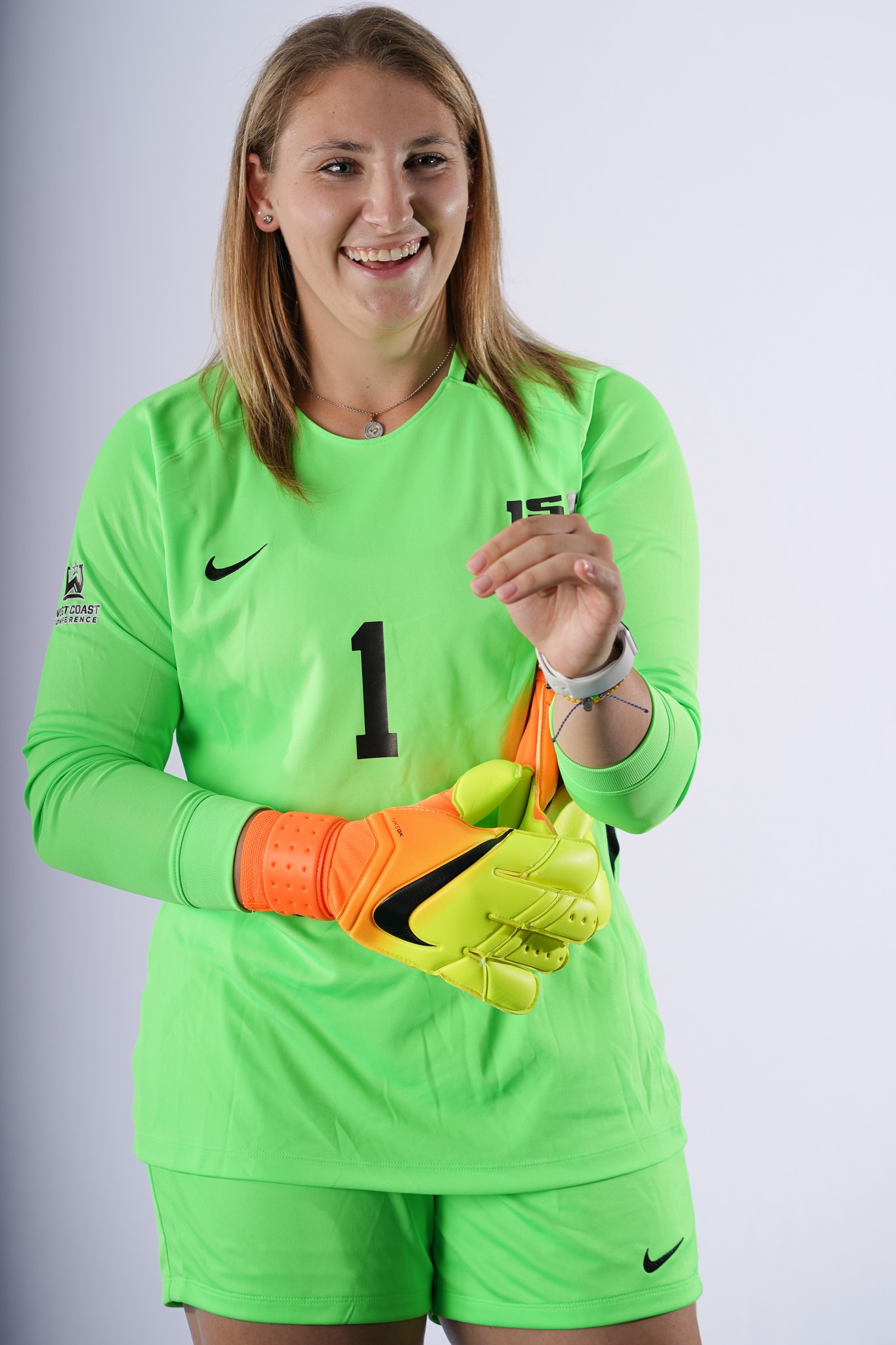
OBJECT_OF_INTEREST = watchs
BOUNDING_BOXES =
[535,617,639,700]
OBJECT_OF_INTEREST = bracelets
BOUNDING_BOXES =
[537,664,649,743]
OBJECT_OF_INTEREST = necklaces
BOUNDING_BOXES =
[299,334,456,439]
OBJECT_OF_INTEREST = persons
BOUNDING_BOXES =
[18,10,707,1345]
